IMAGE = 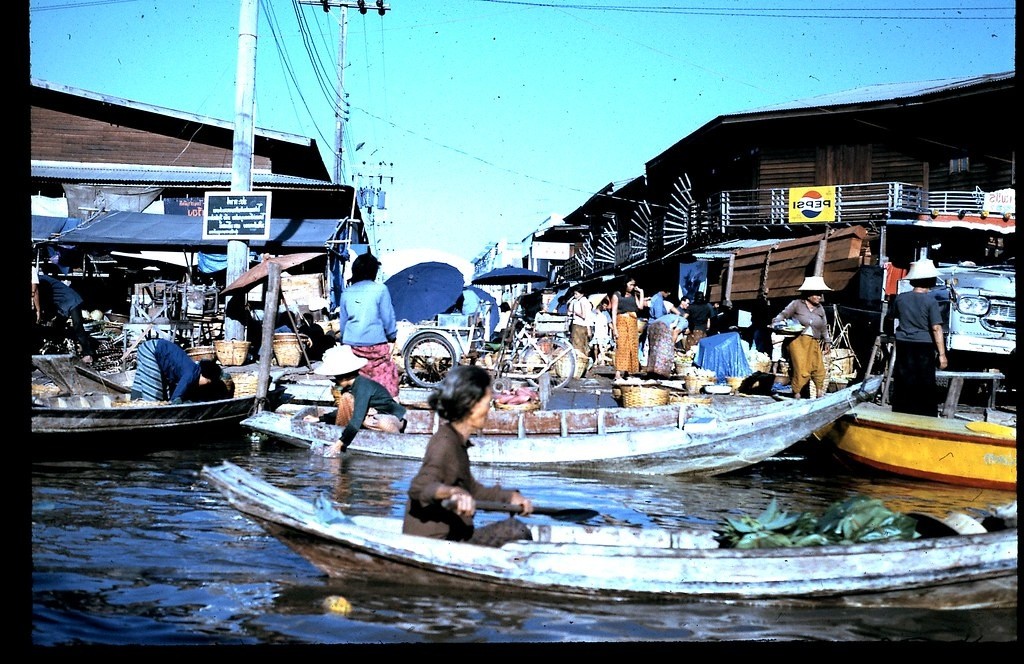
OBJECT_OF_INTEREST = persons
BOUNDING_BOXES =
[130,339,222,402]
[340,254,399,404]
[556,275,566,284]
[402,363,534,548]
[502,289,513,303]
[557,287,614,366]
[32,266,93,364]
[772,276,834,399]
[494,302,512,332]
[893,259,947,417]
[227,293,325,362]
[304,344,406,458]
[612,275,710,382]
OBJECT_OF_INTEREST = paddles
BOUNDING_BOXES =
[441,498,600,522]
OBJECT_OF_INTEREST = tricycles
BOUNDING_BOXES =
[401,295,575,393]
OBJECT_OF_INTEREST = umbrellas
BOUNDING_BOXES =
[471,264,548,310]
[219,253,327,373]
[385,261,465,324]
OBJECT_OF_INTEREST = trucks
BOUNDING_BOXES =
[709,211,1016,403]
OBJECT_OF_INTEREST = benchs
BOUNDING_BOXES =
[935,370,1005,418]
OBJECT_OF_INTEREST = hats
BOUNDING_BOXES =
[797,277,832,291]
[902,259,944,280]
[313,345,369,375]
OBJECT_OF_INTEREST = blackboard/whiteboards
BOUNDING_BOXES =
[202,191,271,240]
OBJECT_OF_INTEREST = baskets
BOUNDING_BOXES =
[273,333,307,366]
[823,348,854,379]
[184,346,217,362]
[668,397,711,406]
[675,358,773,394]
[552,354,590,379]
[215,341,251,366]
[622,387,670,409]
[493,398,542,411]
[231,371,275,399]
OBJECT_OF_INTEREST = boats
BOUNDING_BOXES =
[198,459,1018,609]
[240,374,885,480]
[813,406,1017,491]
[31,394,256,457]
